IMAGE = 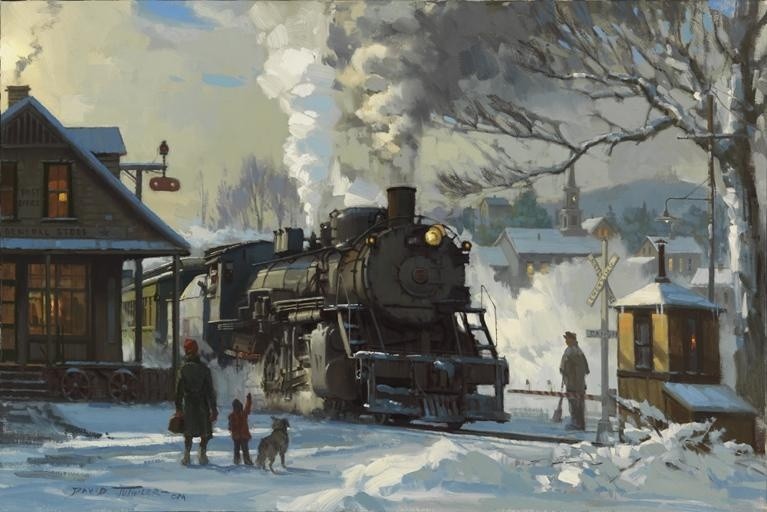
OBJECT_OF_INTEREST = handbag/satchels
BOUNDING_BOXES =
[168,416,185,433]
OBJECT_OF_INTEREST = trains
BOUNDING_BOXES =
[122,187,510,428]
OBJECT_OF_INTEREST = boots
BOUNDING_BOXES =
[199,449,208,466]
[243,452,253,465]
[233,454,240,464]
[181,449,191,464]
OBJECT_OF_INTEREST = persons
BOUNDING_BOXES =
[173,338,219,466]
[228,393,254,466]
[559,331,590,432]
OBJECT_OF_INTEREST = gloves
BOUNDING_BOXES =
[246,394,251,400]
[174,409,181,418]
[209,413,217,423]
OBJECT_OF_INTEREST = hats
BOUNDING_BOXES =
[184,339,198,354]
[563,332,577,339]
[234,400,242,412]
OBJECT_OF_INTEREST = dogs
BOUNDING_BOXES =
[255,416,290,475]
